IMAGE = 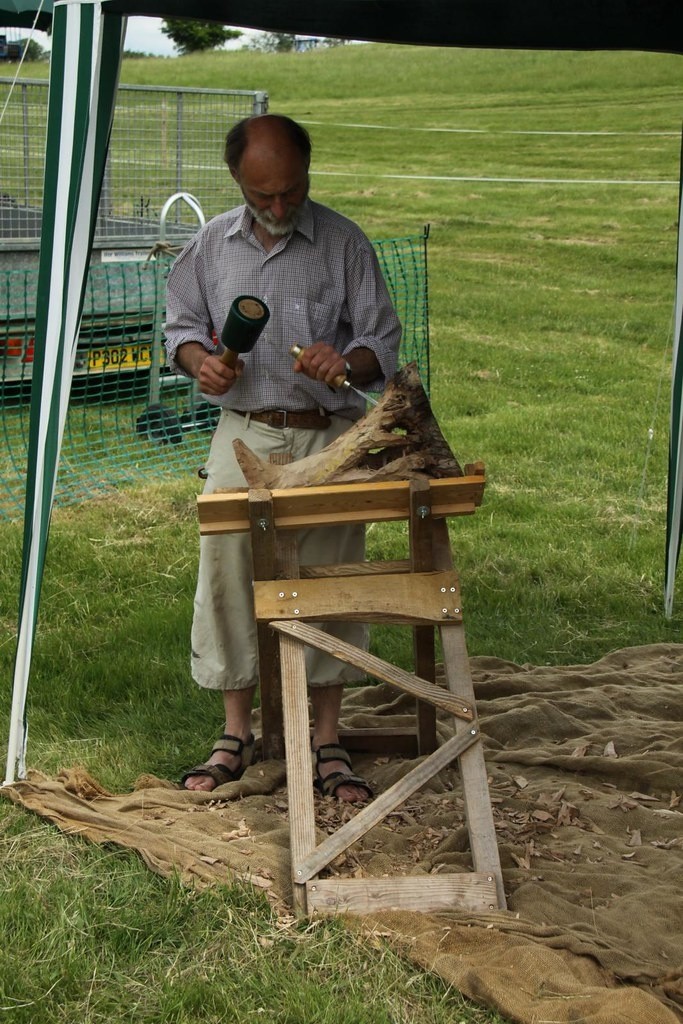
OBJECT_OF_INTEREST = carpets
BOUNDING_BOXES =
[4,637,682,1024]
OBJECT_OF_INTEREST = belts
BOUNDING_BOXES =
[230,409,335,430]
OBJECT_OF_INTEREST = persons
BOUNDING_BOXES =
[165,115,402,808]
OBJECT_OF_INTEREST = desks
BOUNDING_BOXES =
[192,461,511,916]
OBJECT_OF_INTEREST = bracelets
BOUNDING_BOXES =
[345,361,351,377]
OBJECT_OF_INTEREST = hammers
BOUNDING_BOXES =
[218,293,271,368]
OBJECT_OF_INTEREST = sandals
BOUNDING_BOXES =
[312,743,373,806]
[182,733,258,792]
